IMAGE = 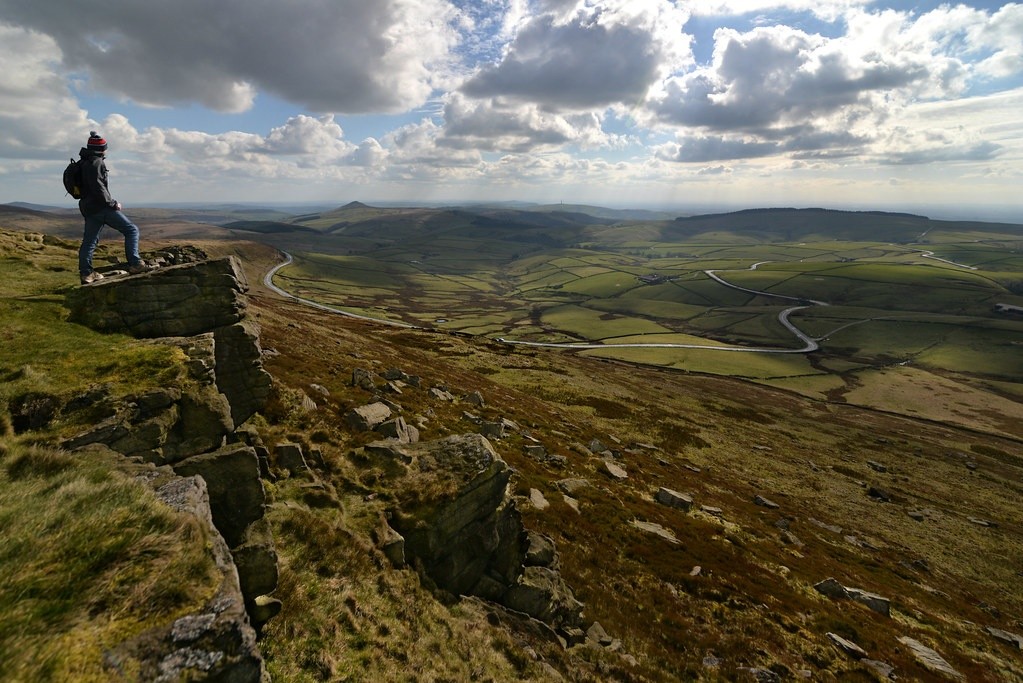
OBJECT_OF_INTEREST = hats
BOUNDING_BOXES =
[87,131,108,152]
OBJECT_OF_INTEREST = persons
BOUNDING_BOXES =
[77,130,160,284]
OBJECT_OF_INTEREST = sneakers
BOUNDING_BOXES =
[80,271,103,284]
[129,260,160,275]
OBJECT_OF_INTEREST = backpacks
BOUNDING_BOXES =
[63,158,86,199]
